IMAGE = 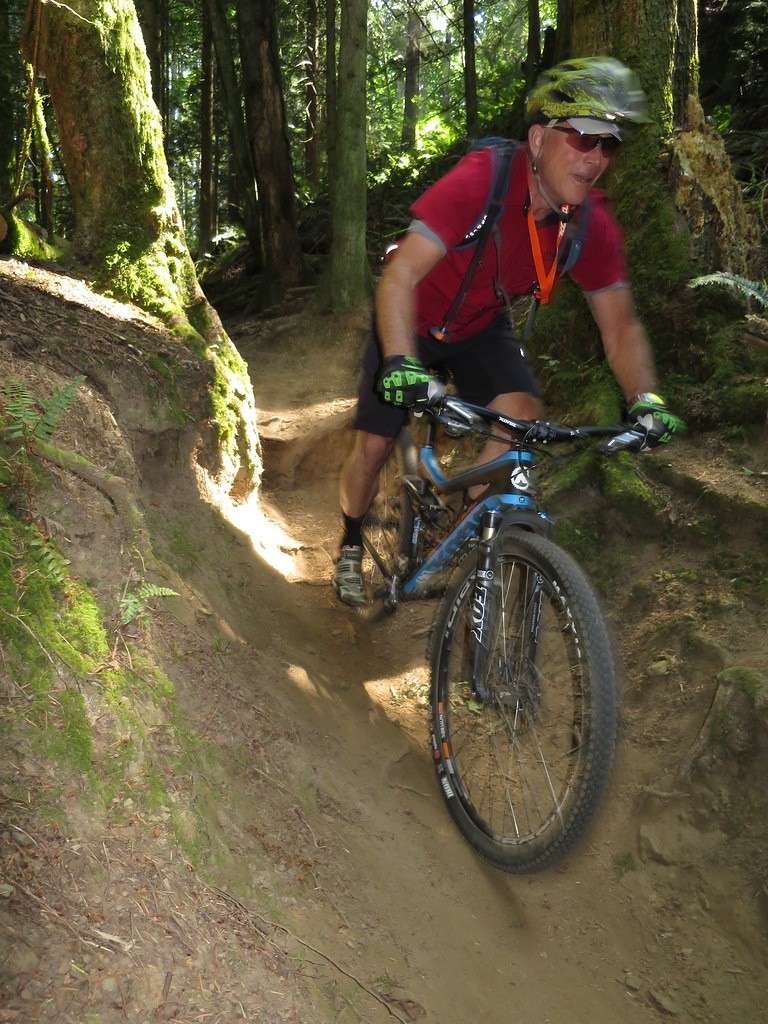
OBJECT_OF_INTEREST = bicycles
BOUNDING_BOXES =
[351,396,640,877]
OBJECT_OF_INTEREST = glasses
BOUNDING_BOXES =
[543,123,622,158]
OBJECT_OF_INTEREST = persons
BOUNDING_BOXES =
[334,55,678,606]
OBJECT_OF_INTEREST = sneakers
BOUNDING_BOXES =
[331,542,370,605]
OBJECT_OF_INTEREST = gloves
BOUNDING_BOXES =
[376,354,446,410]
[627,394,688,454]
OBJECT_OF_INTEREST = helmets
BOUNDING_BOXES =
[524,56,653,128]
